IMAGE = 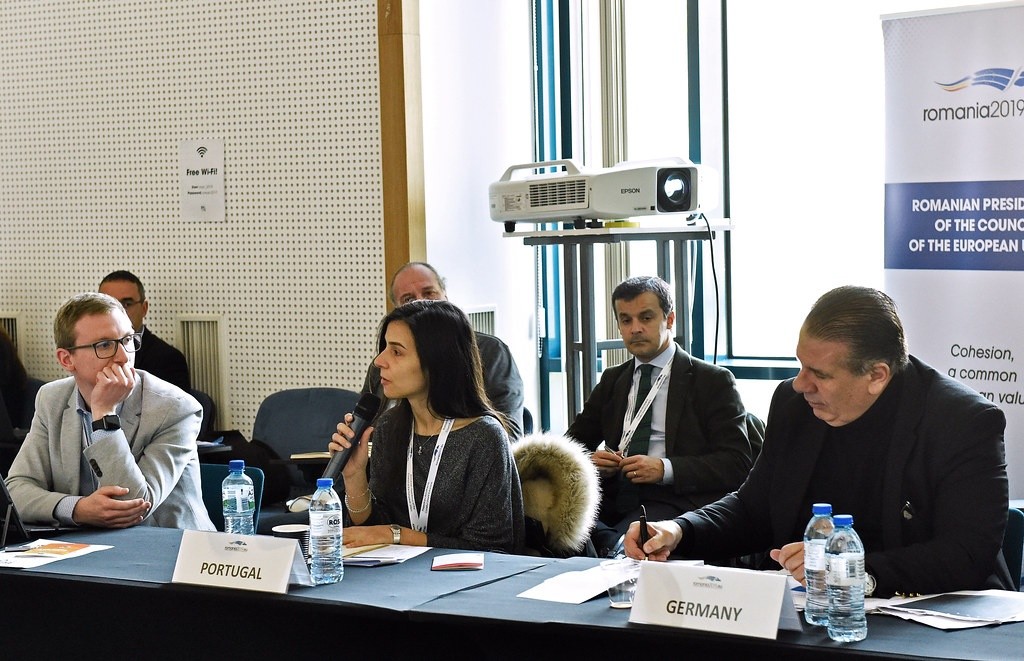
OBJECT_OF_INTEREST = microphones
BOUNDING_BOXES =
[321,393,382,486]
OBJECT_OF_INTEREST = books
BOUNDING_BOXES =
[290,451,332,459]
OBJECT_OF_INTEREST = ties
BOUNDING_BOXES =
[614,364,654,514]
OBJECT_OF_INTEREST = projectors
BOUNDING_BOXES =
[489,159,718,223]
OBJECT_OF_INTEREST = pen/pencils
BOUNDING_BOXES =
[639,505,649,561]
[603,445,624,459]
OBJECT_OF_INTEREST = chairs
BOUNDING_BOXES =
[187,388,1024,591]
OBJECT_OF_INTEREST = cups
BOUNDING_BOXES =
[273,524,309,569]
[600,558,642,609]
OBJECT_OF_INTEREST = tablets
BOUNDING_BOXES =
[1,474,29,542]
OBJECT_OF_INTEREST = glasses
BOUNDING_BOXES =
[64,333,142,359]
[118,299,144,308]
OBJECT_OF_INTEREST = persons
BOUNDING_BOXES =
[361,262,525,442]
[4,291,218,532]
[328,299,524,552]
[0,327,50,428]
[564,275,755,536]
[624,286,1015,600]
[99,270,191,394]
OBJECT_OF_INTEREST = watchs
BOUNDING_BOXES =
[863,572,876,597]
[92,415,121,431]
[390,524,401,544]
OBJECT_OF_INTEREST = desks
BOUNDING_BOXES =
[0,522,1024,661]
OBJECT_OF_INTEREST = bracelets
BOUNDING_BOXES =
[344,490,372,514]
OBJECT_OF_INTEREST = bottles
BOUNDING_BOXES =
[805,503,835,627]
[309,478,342,585]
[825,515,867,643]
[222,460,256,535]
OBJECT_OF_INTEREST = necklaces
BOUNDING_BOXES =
[414,426,442,456]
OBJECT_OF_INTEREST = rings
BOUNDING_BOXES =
[633,471,637,477]
